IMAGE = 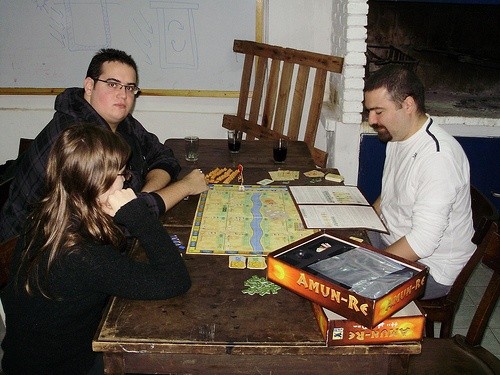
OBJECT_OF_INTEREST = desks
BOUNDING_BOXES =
[92,227,422,375]
[160,167,366,230]
[164,139,315,167]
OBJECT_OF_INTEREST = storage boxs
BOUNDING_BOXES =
[268,230,430,348]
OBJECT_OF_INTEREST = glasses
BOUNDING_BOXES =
[116,169,132,181]
[92,77,141,95]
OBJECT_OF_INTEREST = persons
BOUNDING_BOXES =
[0,48,210,293]
[0,123,191,375]
[363,63,478,303]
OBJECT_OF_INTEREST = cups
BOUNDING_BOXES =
[272,135,288,163]
[184,135,199,161]
[227,129,242,154]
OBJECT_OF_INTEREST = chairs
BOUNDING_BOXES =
[0,233,21,301]
[422,185,498,338]
[407,233,500,375]
